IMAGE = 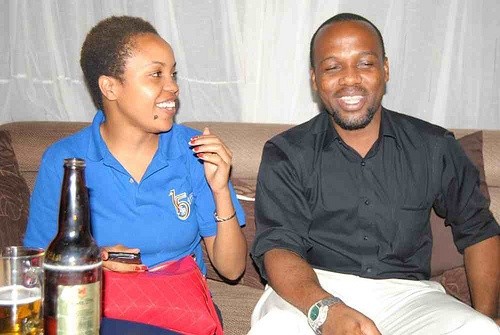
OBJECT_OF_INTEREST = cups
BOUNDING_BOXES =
[0,246,47,335]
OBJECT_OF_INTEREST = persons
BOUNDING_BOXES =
[22,13,246,284]
[252,11,499,335]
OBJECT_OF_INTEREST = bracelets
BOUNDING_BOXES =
[213,208,236,222]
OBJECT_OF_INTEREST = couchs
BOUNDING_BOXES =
[0,122,500,335]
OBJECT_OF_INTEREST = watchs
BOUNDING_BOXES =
[307,296,345,335]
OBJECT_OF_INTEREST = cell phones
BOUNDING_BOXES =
[107,252,142,265]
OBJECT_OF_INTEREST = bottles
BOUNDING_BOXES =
[44,158,104,335]
